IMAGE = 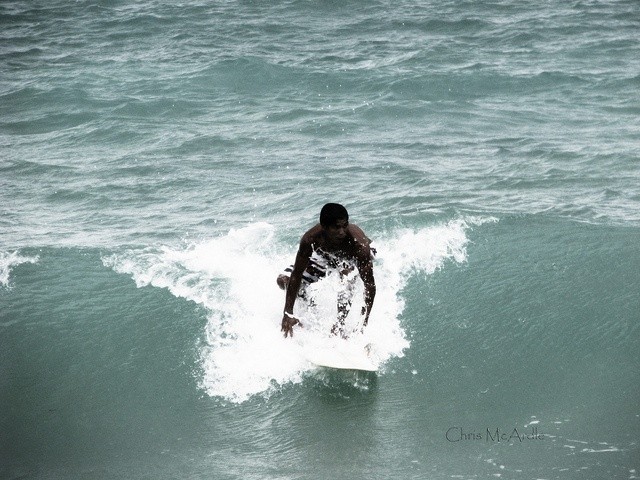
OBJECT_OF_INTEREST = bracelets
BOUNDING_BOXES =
[284,311,300,320]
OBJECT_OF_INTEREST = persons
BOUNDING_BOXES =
[274,202,377,338]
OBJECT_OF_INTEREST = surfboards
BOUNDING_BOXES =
[311,336,379,371]
